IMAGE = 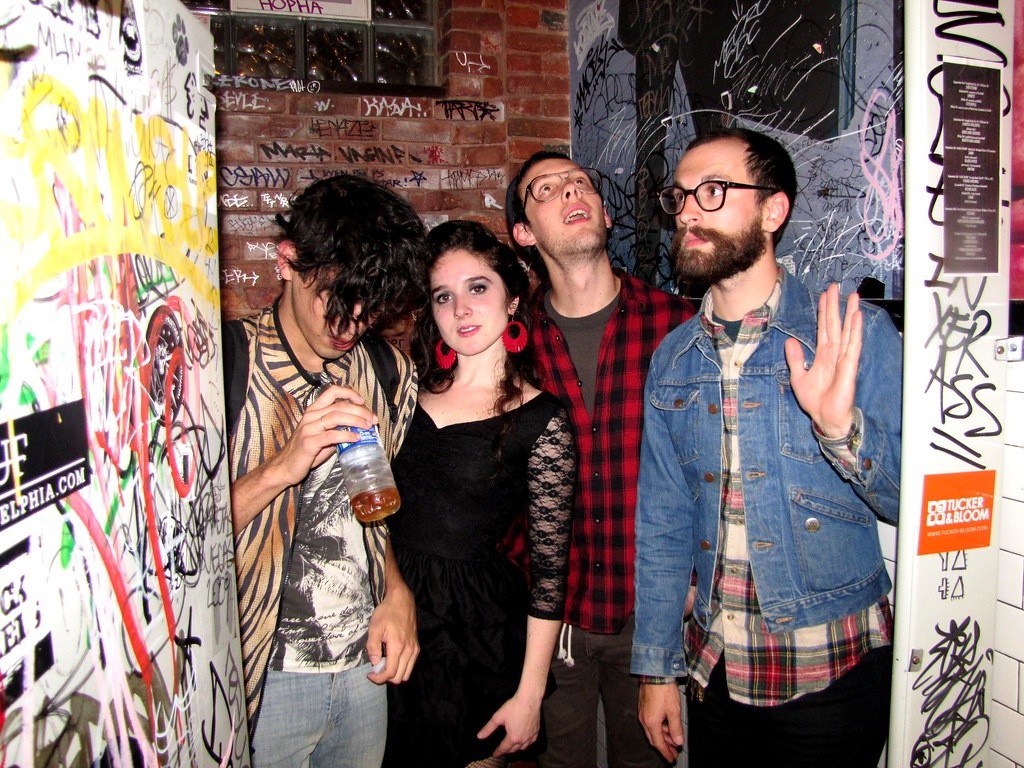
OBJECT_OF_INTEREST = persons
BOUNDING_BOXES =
[382,221,579,768]
[505,150,699,768]
[625,127,904,768]
[224,175,430,768]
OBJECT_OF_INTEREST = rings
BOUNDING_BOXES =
[321,416,327,431]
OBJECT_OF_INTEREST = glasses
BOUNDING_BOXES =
[656,179,780,215]
[522,167,603,220]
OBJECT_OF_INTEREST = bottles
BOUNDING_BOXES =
[331,386,400,523]
